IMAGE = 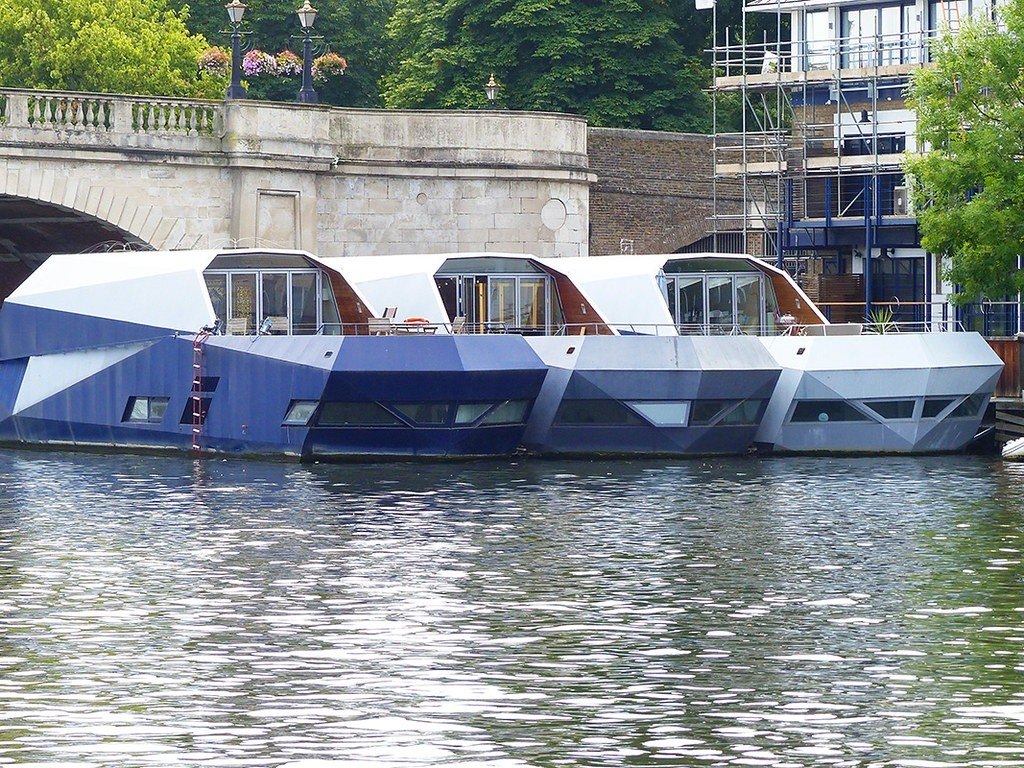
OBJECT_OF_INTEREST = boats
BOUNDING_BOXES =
[2,242,1010,460]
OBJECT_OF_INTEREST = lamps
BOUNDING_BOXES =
[875,247,895,261]
[260,318,271,335]
[483,73,501,108]
[858,109,872,125]
[853,250,863,259]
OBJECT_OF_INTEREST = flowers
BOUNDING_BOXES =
[198,50,347,82]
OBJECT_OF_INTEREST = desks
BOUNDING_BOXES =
[392,326,438,335]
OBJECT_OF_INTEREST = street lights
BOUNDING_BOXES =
[216,0,254,101]
[287,0,325,103]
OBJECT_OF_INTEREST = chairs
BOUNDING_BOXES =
[227,316,288,335]
[802,324,862,336]
[367,307,466,335]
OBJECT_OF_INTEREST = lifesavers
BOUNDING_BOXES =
[404,318,430,325]
